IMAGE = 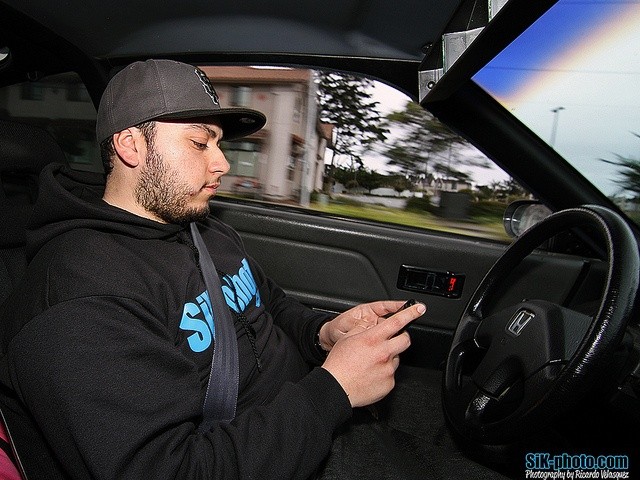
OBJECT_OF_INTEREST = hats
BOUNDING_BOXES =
[96,59,266,148]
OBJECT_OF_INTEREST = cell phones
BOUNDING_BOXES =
[386,299,418,321]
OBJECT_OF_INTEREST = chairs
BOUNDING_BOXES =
[0,119,94,480]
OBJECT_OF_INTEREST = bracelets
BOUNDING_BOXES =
[314,318,331,356]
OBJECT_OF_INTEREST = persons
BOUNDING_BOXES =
[10,57,435,474]
